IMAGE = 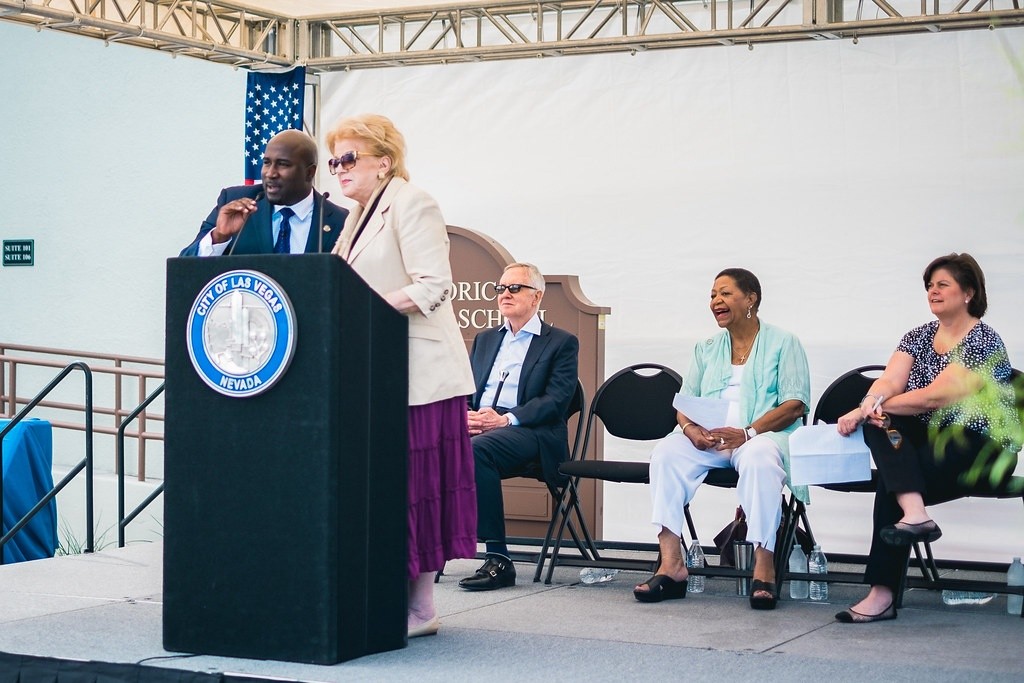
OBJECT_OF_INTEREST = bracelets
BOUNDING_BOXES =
[508,419,512,426]
[861,395,877,403]
[743,428,747,442]
[683,423,695,434]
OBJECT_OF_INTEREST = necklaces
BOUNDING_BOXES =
[732,324,759,362]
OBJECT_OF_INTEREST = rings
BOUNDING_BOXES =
[721,438,724,444]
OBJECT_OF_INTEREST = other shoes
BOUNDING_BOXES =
[880,519,942,545]
[407,614,439,638]
[836,599,897,623]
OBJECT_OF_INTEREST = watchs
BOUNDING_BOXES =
[744,426,757,439]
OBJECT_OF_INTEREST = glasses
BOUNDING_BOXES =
[495,284,539,296]
[328,152,376,175]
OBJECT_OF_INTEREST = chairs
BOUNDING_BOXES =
[433,363,1024,618]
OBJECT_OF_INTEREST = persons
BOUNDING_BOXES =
[459,263,580,591]
[179,130,349,257]
[326,113,478,637]
[835,253,1022,622]
[634,268,810,609]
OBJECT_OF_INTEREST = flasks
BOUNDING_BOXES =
[734,540,753,596]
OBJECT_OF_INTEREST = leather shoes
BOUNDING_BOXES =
[459,559,515,591]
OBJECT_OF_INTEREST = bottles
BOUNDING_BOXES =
[943,590,997,605]
[687,539,704,593]
[789,545,808,600]
[1007,558,1024,614]
[580,567,618,584]
[809,545,828,601]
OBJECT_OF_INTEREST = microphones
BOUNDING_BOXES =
[230,192,265,255]
[319,192,330,253]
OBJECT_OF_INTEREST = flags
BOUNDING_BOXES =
[245,66,307,186]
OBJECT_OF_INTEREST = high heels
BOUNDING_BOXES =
[634,574,688,602]
[749,580,777,610]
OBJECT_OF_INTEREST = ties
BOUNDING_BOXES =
[274,208,294,255]
